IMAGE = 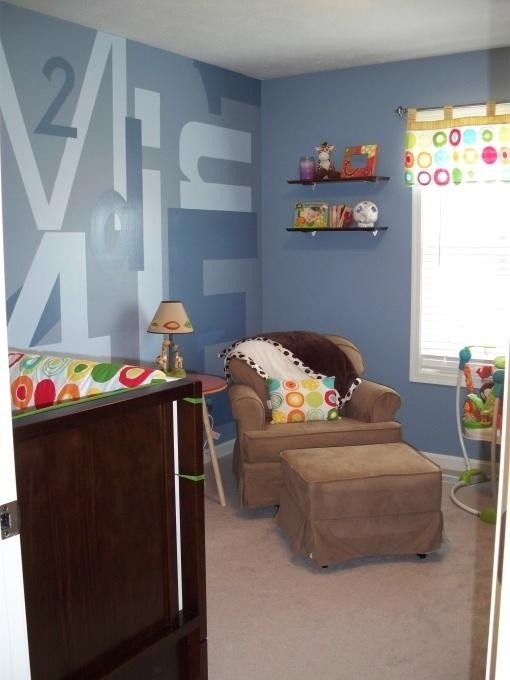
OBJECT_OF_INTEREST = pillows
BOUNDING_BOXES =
[265,374,341,425]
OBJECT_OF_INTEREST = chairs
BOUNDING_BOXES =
[222,329,406,511]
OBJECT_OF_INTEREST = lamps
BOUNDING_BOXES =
[147,299,195,379]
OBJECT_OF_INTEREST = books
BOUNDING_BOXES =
[328,204,354,231]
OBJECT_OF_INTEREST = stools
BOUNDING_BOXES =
[271,441,446,570]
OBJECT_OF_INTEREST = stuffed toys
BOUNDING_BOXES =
[314,142,340,180]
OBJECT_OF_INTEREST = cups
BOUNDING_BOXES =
[299,156,315,180]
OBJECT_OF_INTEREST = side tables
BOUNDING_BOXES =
[169,372,229,509]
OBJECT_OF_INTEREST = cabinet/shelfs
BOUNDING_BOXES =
[286,174,390,235]
